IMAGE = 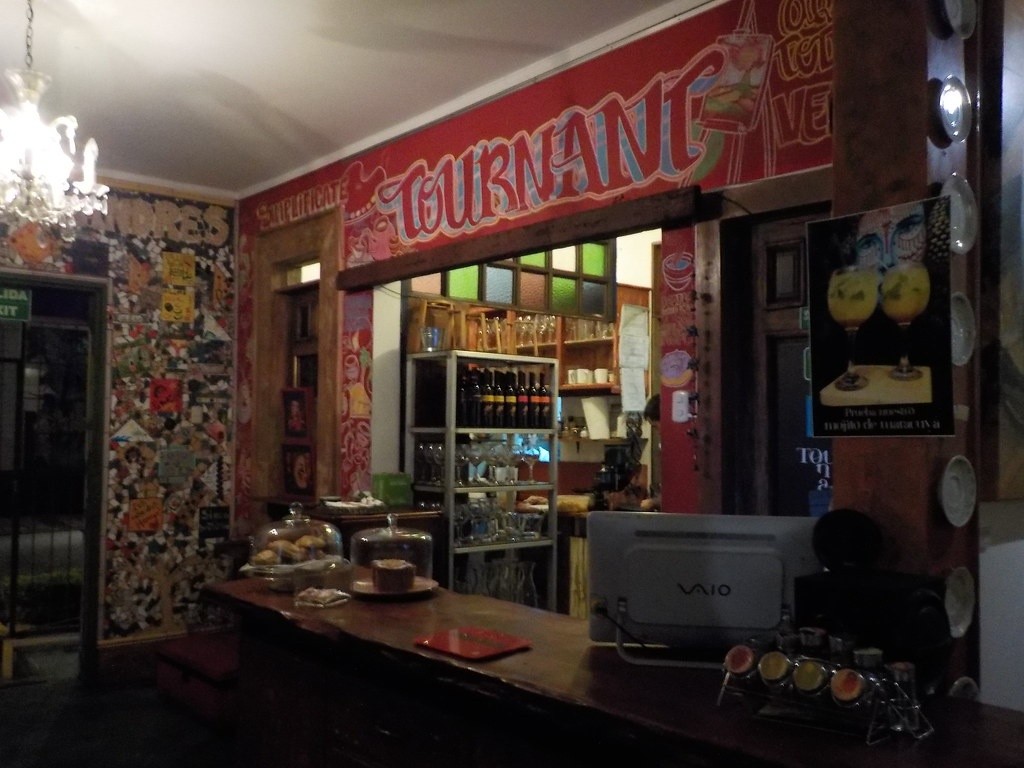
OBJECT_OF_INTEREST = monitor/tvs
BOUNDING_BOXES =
[585,511,825,647]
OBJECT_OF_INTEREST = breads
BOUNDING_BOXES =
[371,558,416,592]
[268,535,328,561]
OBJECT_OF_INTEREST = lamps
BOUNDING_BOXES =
[0,0,111,246]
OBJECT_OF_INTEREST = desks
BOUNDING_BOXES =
[202,571,1024,768]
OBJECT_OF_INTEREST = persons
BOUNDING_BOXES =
[639,392,661,512]
[851,200,929,304]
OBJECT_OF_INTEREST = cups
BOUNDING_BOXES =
[568,367,613,384]
[420,326,447,352]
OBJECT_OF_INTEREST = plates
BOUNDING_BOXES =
[350,576,440,598]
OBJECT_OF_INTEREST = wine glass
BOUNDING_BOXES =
[416,442,540,489]
[416,497,545,544]
[477,316,616,349]
[882,261,930,382]
[827,263,878,391]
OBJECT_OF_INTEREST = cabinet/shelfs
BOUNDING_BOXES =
[405,349,557,610]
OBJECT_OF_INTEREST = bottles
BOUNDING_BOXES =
[456,366,551,428]
